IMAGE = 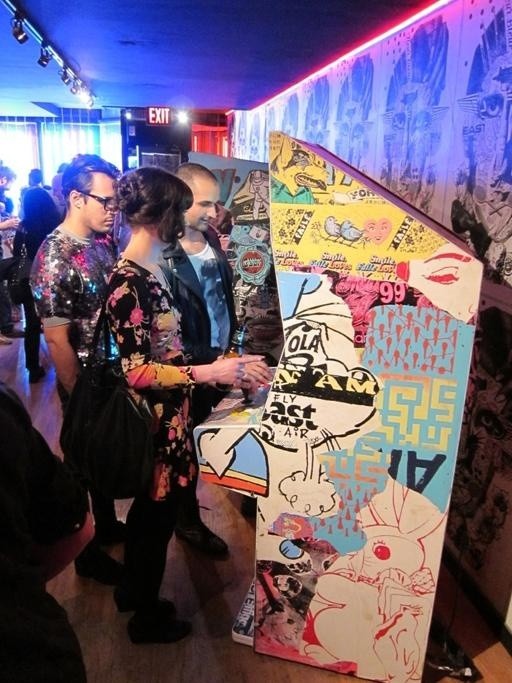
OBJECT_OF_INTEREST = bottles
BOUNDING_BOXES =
[214,324,245,393]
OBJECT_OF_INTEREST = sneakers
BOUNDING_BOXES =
[113,586,192,644]
[74,521,124,586]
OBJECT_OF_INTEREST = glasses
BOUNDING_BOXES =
[75,189,118,211]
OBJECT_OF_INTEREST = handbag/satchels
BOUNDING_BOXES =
[58,276,150,497]
[9,243,32,305]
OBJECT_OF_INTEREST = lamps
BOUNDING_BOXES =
[9,11,82,95]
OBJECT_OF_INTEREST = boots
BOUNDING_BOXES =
[175,501,227,557]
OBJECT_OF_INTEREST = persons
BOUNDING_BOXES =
[0,379,97,683]
[29,153,127,588]
[158,161,274,559]
[1,161,68,382]
[109,165,264,645]
